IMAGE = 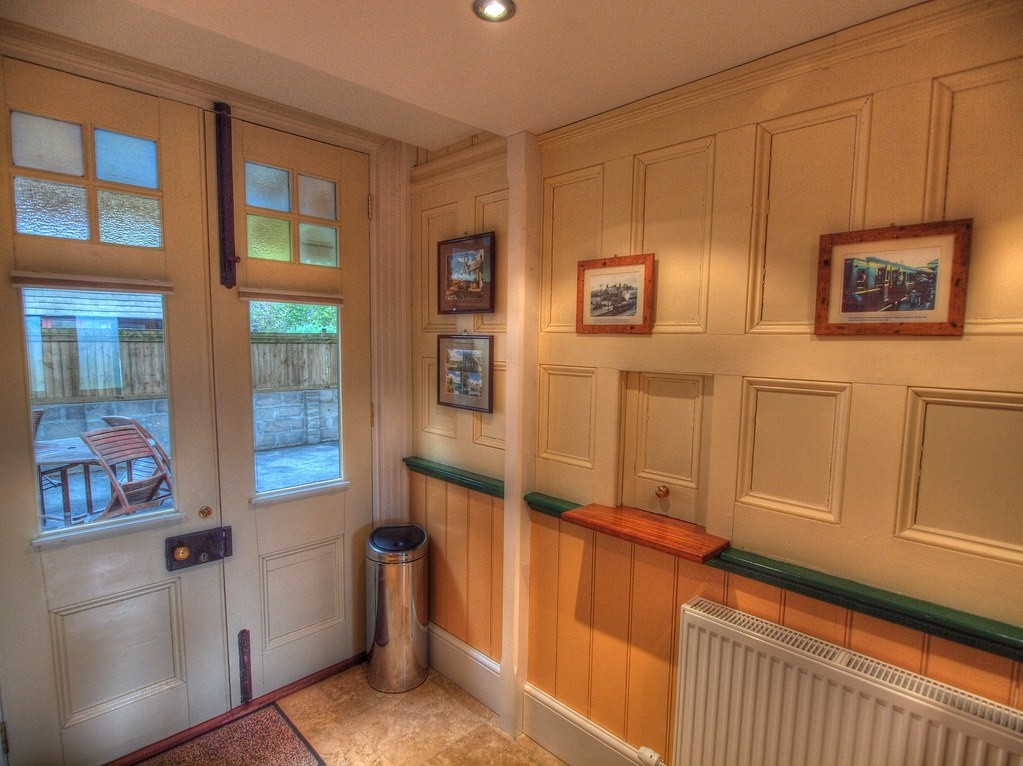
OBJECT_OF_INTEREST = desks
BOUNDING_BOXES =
[34,437,156,528]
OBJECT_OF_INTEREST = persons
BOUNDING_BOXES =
[891,273,936,310]
[454,350,481,395]
[609,287,635,315]
[857,273,866,286]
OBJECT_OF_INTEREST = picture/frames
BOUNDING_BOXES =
[812,215,972,337]
[436,230,495,313]
[574,252,658,334]
[436,335,494,413]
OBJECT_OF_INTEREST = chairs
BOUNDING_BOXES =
[30,408,174,531]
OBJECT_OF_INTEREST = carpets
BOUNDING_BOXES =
[125,702,329,766]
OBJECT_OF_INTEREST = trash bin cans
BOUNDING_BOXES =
[363,525,434,693]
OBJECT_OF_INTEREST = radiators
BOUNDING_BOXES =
[668,595,1023,766]
